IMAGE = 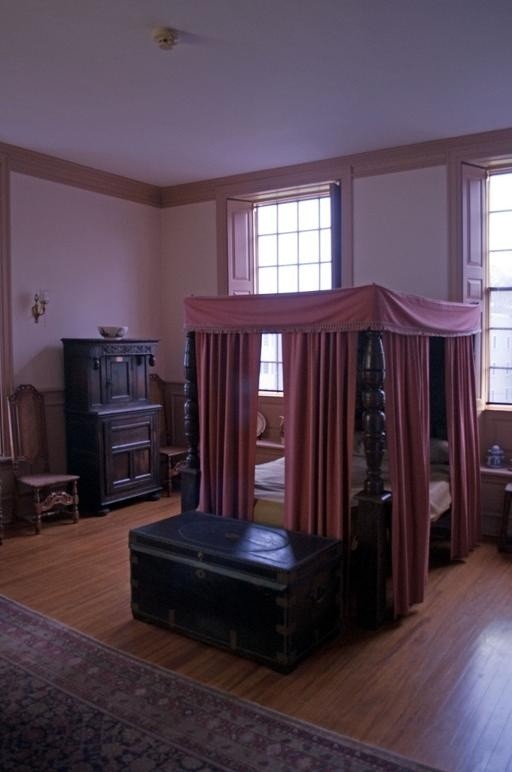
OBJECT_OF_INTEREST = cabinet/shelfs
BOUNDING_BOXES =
[60,338,164,516]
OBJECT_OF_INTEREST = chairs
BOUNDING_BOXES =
[146,374,187,498]
[5,382,81,535]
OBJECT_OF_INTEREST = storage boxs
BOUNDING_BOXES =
[129,510,345,675]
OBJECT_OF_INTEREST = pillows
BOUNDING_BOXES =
[351,430,366,458]
[430,438,449,465]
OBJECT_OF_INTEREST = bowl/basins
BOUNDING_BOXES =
[96,325,128,340]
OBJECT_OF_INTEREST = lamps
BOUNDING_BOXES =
[152,25,177,52]
[31,289,49,324]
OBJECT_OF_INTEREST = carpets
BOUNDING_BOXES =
[1,595,448,772]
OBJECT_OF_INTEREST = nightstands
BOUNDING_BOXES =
[475,463,512,540]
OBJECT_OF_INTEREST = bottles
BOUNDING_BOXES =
[485,445,505,468]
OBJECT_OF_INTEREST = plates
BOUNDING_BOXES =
[256,410,266,438]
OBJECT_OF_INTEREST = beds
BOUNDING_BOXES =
[186,421,463,571]
